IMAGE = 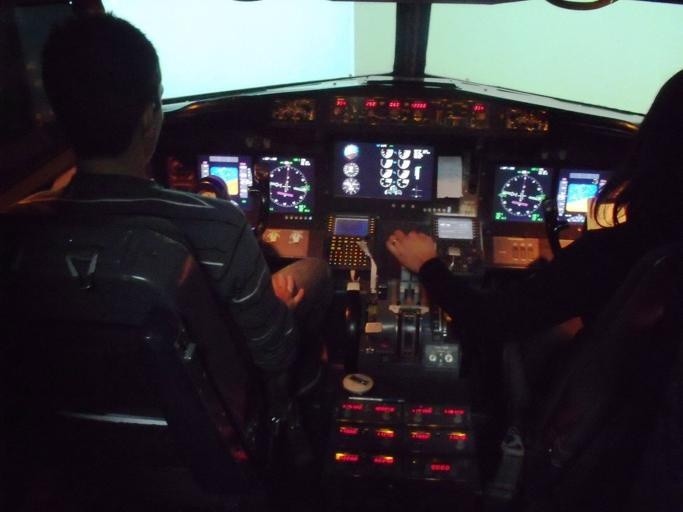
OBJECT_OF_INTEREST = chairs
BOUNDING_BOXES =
[2,218,259,510]
[516,232,679,510]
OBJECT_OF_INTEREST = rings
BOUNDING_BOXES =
[392,239,398,246]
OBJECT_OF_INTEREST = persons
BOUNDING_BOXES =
[384,69,683,452]
[1,12,336,470]
